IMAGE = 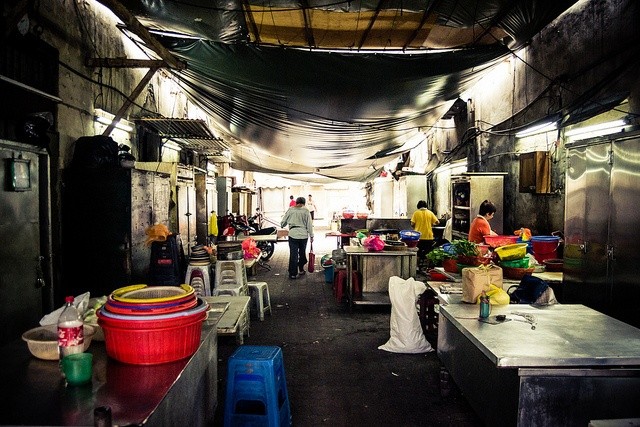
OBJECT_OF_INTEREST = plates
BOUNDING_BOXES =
[188,244,211,266]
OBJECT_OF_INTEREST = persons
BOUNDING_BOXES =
[410,200,440,255]
[280,197,314,280]
[306,194,317,223]
[290,195,295,207]
[468,199,498,245]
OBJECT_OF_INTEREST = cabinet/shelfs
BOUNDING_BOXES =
[451,172,509,241]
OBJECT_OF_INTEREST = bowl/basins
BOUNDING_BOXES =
[22,324,98,360]
[399,230,421,241]
[404,240,419,246]
[503,266,535,280]
[500,256,529,268]
[476,245,489,253]
[92,278,210,366]
[540,258,563,272]
[442,257,459,273]
[342,211,354,218]
[460,255,496,269]
[357,212,368,219]
[482,234,521,247]
[495,243,528,260]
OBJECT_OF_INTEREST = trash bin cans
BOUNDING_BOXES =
[321,258,336,283]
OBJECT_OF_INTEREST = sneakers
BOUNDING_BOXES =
[299,270,305,275]
[289,275,296,279]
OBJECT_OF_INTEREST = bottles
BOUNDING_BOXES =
[58,296,85,361]
[479,290,491,318]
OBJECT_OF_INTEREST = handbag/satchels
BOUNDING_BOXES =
[308,243,315,273]
[507,276,548,303]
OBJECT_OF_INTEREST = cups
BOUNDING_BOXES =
[60,353,92,387]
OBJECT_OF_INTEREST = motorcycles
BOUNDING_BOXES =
[217,213,277,259]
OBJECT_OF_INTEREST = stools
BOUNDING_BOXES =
[213,259,250,344]
[184,263,212,296]
[148,233,184,287]
[225,345,291,426]
[248,281,272,321]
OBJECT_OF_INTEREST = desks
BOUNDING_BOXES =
[427,281,540,304]
[0,301,231,427]
[239,235,277,272]
[437,303,639,426]
[343,249,425,314]
[326,232,356,249]
